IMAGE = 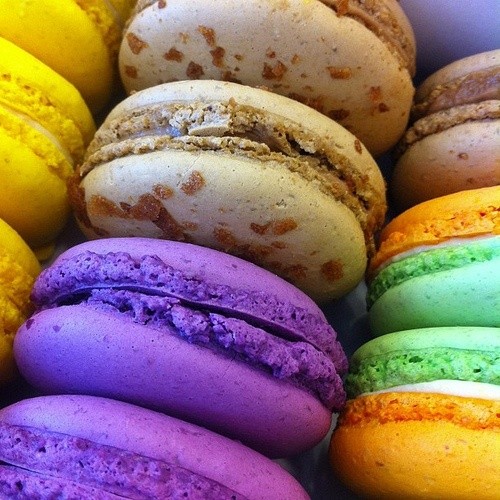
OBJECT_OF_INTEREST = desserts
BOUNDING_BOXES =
[0,2,500,500]
